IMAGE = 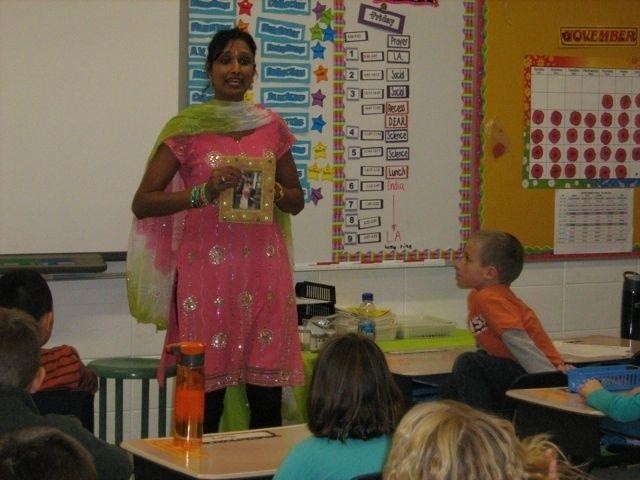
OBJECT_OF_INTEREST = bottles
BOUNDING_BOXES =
[164,341,206,454]
[620,269,640,341]
[358,293,377,345]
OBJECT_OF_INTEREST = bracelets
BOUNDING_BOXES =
[188,183,211,209]
[274,181,285,204]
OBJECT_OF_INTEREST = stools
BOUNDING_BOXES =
[87,357,178,445]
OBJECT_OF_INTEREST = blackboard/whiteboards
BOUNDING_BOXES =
[0,0,189,274]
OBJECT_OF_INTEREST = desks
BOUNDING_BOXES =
[117,422,313,480]
[548,331,638,368]
[505,385,640,465]
[387,334,479,413]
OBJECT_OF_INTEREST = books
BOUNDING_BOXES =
[333,306,396,327]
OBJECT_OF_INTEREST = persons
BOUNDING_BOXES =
[131,28,304,434]
[381,396,596,480]
[237,172,253,209]
[1,424,96,479]
[0,267,100,432]
[450,229,575,419]
[578,377,640,425]
[273,331,406,480]
[1,304,137,480]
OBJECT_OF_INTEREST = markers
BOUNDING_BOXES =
[309,261,340,266]
[395,259,425,262]
[352,259,382,263]
